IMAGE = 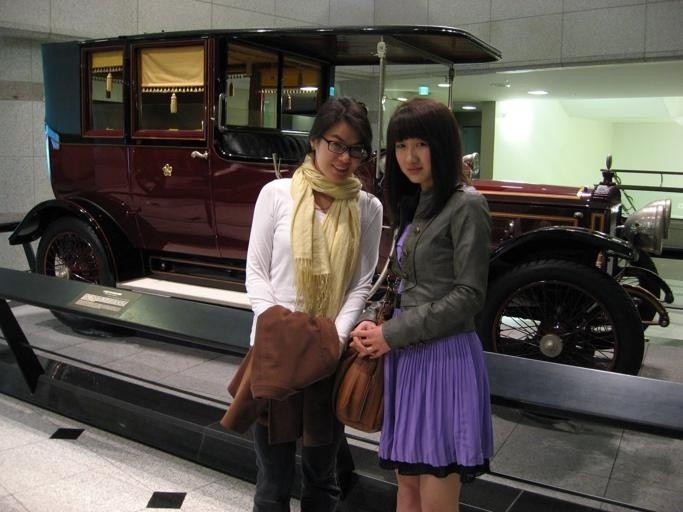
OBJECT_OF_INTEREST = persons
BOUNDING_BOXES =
[348,96,494,512]
[244,95,385,512]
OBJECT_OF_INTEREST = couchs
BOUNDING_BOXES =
[215,125,310,157]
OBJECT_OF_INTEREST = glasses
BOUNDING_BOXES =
[320,136,368,158]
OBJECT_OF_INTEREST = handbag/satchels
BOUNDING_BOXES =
[337,303,389,433]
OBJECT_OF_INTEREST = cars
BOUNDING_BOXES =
[7,24,673,379]
